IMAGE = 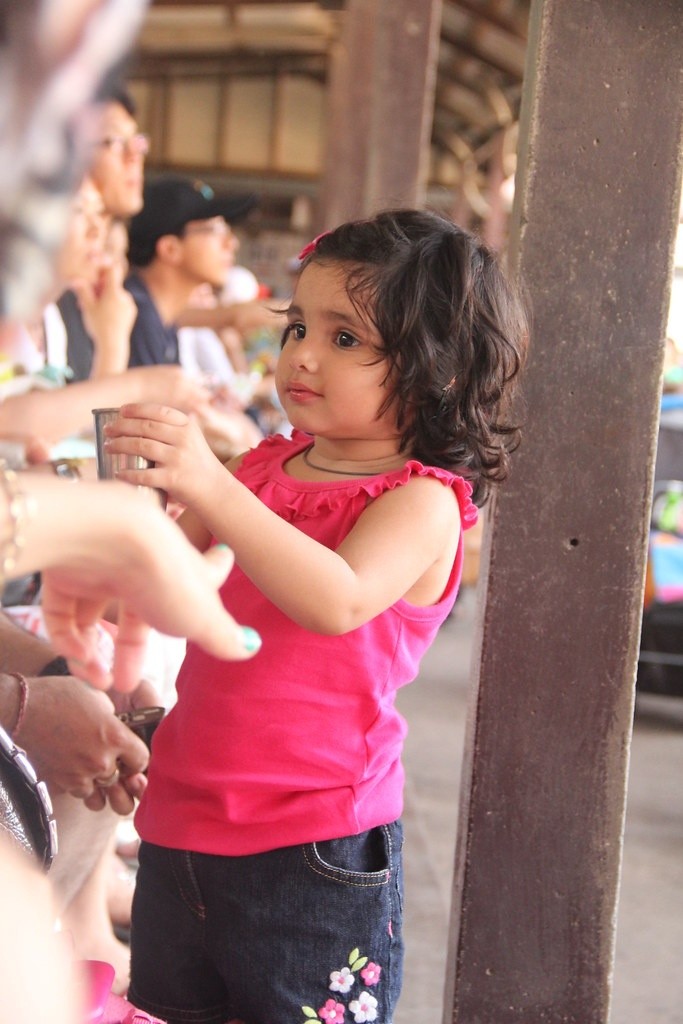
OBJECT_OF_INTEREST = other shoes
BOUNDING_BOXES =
[75,960,168,1024]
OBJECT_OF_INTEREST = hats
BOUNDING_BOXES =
[125,169,258,266]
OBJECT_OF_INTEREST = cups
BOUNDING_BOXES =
[92,408,167,512]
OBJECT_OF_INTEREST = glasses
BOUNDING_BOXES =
[75,132,151,158]
[179,220,229,239]
[72,203,115,230]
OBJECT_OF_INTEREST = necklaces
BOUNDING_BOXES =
[303,444,381,478]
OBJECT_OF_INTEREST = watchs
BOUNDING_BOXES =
[50,458,78,485]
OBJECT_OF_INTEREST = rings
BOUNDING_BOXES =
[95,768,120,788]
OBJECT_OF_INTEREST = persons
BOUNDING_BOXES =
[0,0,299,1024]
[96,208,528,1024]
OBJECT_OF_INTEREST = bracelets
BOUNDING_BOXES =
[6,671,29,743]
[1,460,23,578]
